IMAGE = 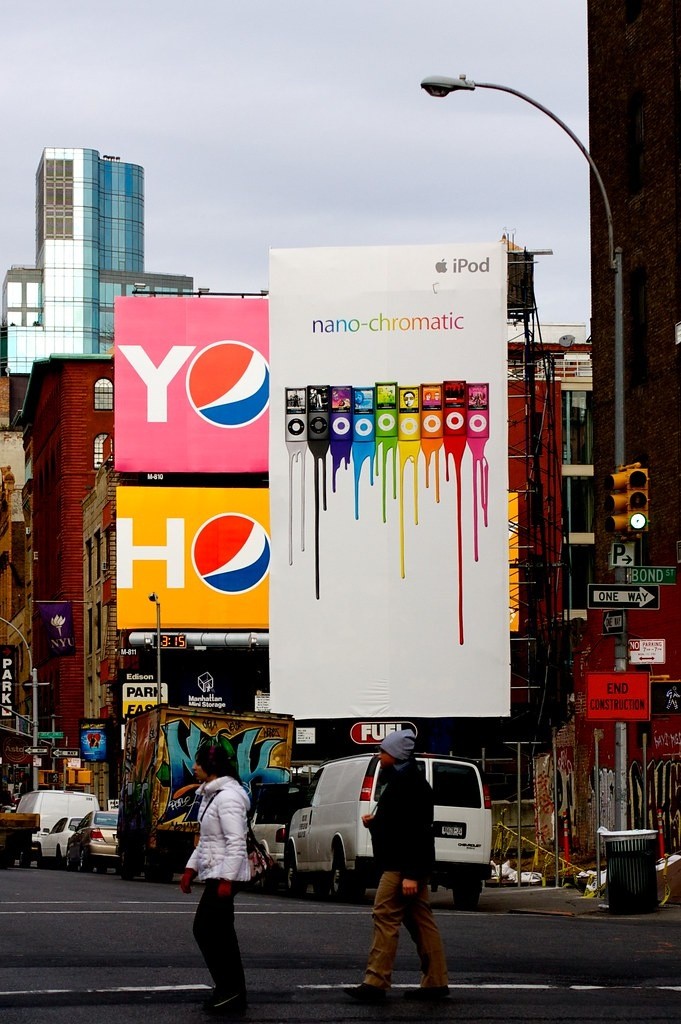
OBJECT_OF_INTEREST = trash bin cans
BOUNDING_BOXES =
[597,829,659,915]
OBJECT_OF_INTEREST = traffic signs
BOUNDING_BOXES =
[602,610,626,635]
[627,638,666,665]
[50,747,80,758]
[587,583,660,610]
[24,745,49,756]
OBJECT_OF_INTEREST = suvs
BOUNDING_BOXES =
[248,781,307,893]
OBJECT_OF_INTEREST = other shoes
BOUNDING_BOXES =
[404,985,448,1001]
[342,981,386,1004]
[206,985,246,1009]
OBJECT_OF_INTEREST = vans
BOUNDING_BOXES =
[284,751,494,909]
[16,790,100,866]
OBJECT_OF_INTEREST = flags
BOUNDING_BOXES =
[40,604,77,658]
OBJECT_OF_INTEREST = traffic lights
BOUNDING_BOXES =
[604,461,651,541]
[44,770,64,785]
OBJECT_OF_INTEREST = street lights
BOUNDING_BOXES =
[149,592,162,704]
[421,72,630,831]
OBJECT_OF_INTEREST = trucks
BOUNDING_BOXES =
[117,703,294,883]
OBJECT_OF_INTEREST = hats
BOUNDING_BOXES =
[379,729,415,760]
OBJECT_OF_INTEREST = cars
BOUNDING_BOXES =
[37,810,120,872]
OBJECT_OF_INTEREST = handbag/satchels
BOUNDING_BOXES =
[245,836,275,882]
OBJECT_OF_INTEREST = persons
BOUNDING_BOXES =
[2,781,11,795]
[180,745,251,1008]
[342,729,450,1003]
